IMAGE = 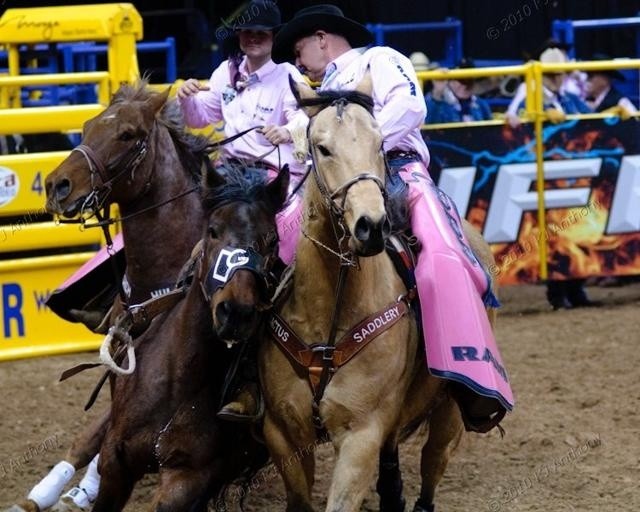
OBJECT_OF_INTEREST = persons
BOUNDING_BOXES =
[67,1,639,419]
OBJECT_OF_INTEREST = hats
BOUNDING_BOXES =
[221,1,282,30]
[540,47,627,84]
[271,5,371,64]
[409,51,439,71]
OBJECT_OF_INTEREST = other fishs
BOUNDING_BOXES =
[4,70,498,512]
